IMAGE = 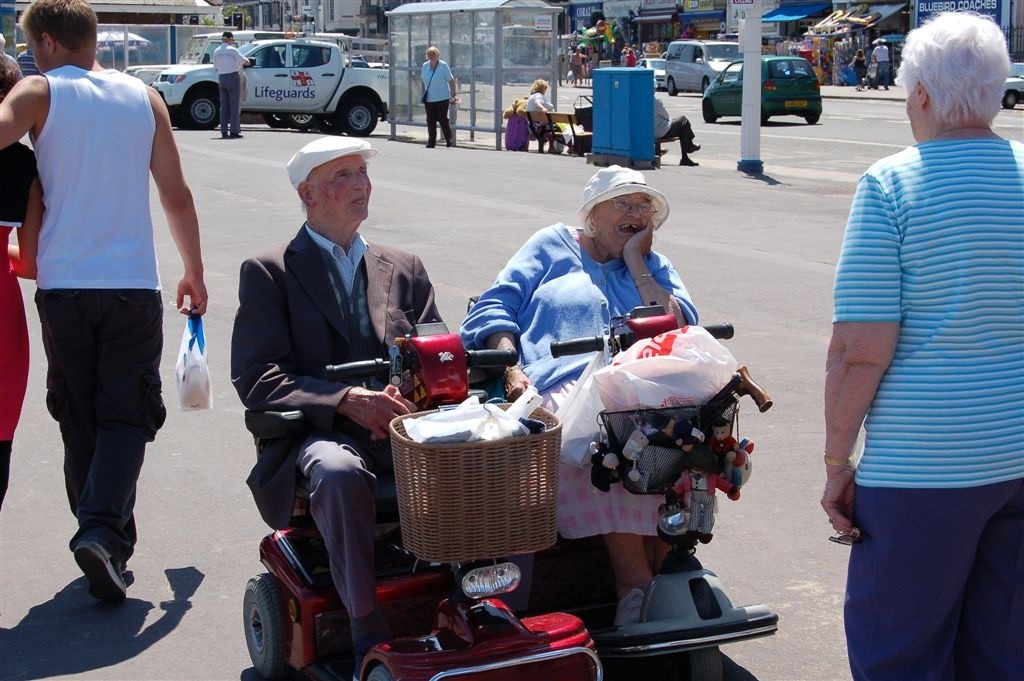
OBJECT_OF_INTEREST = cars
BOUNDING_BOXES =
[1001,62,1024,110]
[635,58,667,91]
[702,55,822,126]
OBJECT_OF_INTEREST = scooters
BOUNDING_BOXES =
[244,301,781,681]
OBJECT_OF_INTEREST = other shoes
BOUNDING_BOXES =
[425,143,435,148]
[687,144,700,153]
[230,135,243,138]
[680,158,699,166]
[446,138,453,146]
[614,589,645,631]
[222,135,230,138]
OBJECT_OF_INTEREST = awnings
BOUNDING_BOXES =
[761,4,831,22]
[803,2,908,38]
[632,10,724,23]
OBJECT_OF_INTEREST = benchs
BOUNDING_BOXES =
[523,111,681,169]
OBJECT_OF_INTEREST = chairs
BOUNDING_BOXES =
[265,51,280,67]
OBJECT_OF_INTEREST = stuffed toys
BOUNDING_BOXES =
[503,97,530,118]
[589,414,752,542]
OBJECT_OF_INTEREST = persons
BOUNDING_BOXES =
[421,46,456,148]
[0,0,209,600]
[526,79,563,154]
[213,31,250,137]
[570,48,588,84]
[620,43,642,67]
[459,164,699,626]
[654,78,701,166]
[847,40,890,90]
[230,137,535,681]
[820,10,1024,681]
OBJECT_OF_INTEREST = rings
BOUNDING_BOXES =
[829,520,833,523]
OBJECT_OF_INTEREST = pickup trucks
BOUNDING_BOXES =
[150,38,414,138]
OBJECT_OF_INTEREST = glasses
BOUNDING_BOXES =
[607,196,658,219]
[828,527,860,547]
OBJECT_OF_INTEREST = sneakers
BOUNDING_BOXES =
[72,542,128,603]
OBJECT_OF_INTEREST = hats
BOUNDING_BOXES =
[286,135,378,193]
[222,31,235,41]
[872,37,887,44]
[575,164,671,232]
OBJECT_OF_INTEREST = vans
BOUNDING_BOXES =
[664,39,744,97]
[415,40,517,86]
[120,30,346,86]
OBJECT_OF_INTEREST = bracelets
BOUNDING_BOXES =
[632,273,653,280]
[824,456,849,465]
[636,281,652,288]
[504,364,520,376]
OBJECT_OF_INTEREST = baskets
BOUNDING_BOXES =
[388,402,564,564]
[600,399,739,496]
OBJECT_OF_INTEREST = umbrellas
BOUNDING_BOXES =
[872,33,906,75]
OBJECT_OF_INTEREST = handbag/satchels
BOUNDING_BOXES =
[555,335,614,473]
[591,321,736,480]
[400,386,546,446]
[420,90,428,103]
[174,305,213,413]
